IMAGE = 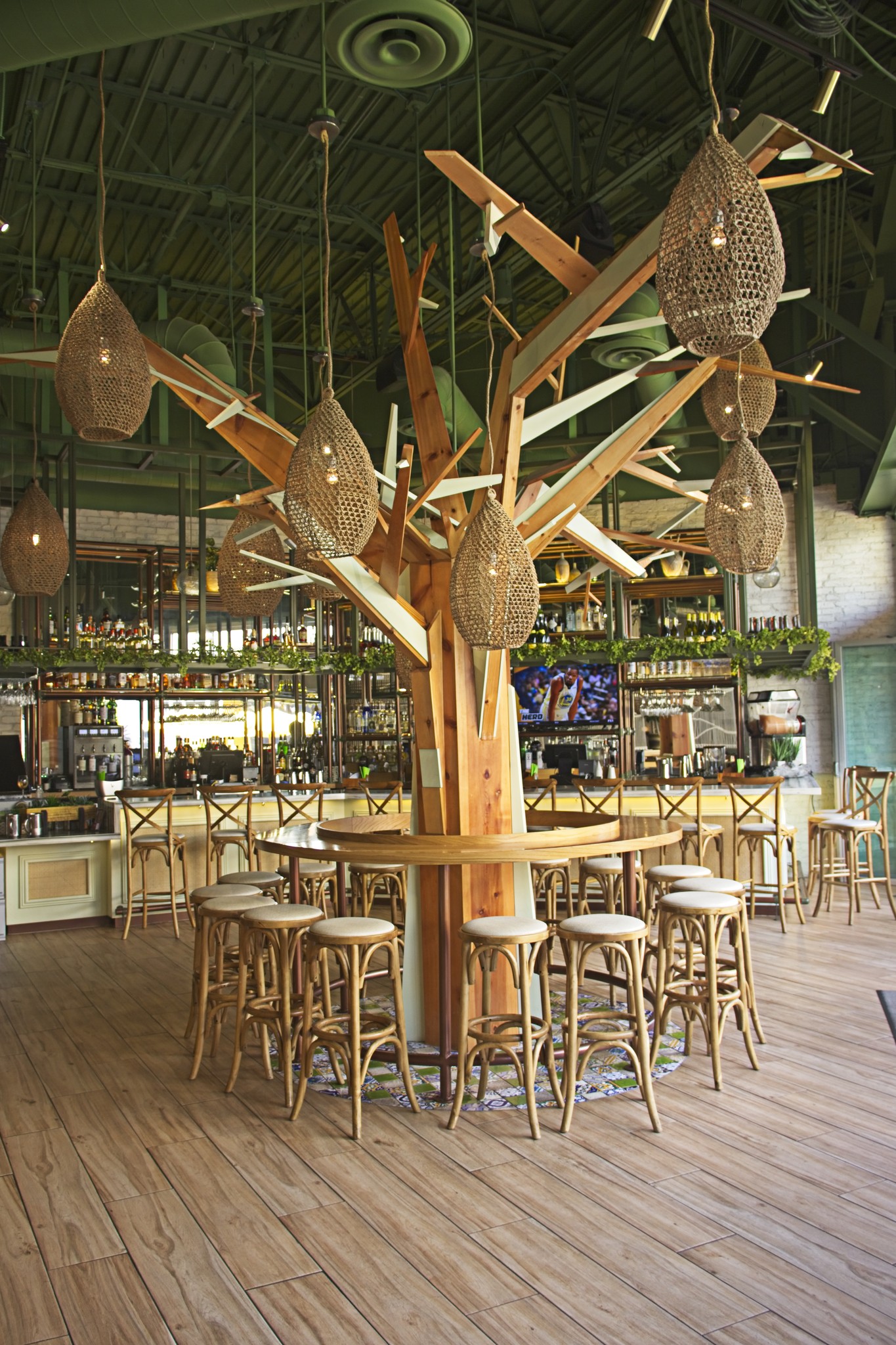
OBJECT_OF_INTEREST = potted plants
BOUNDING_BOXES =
[767,732,801,768]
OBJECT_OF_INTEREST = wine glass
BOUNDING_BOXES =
[0,680,35,705]
[124,764,147,788]
[158,705,246,722]
[17,775,28,795]
[639,692,724,716]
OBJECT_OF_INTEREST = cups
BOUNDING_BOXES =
[657,746,725,778]
[24,813,43,838]
[6,813,18,837]
[608,766,617,779]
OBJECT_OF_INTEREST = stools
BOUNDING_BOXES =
[115,765,892,1139]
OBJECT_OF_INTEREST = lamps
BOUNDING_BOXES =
[704,348,786,575]
[449,0,541,651]
[53,48,152,443]
[656,0,787,358]
[218,62,286,617]
[1,115,69,598]
[701,339,774,442]
[284,0,379,561]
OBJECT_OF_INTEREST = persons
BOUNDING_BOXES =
[540,665,583,721]
[514,663,618,722]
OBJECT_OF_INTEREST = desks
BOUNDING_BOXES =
[254,810,682,1065]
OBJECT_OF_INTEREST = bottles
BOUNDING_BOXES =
[48,605,152,649]
[43,672,255,690]
[0,635,29,652]
[748,615,804,644]
[345,613,392,657]
[662,613,726,642]
[555,552,589,583]
[661,548,690,577]
[527,604,615,644]
[348,703,409,776]
[244,629,257,650]
[43,767,51,790]
[276,679,307,697]
[263,616,307,647]
[155,735,198,787]
[521,735,620,767]
[78,756,117,775]
[72,696,118,725]
[242,744,257,766]
[627,658,731,678]
[276,734,325,784]
[198,736,237,751]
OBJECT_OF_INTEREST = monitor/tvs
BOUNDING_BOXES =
[545,743,588,785]
[200,750,244,785]
[511,663,620,730]
